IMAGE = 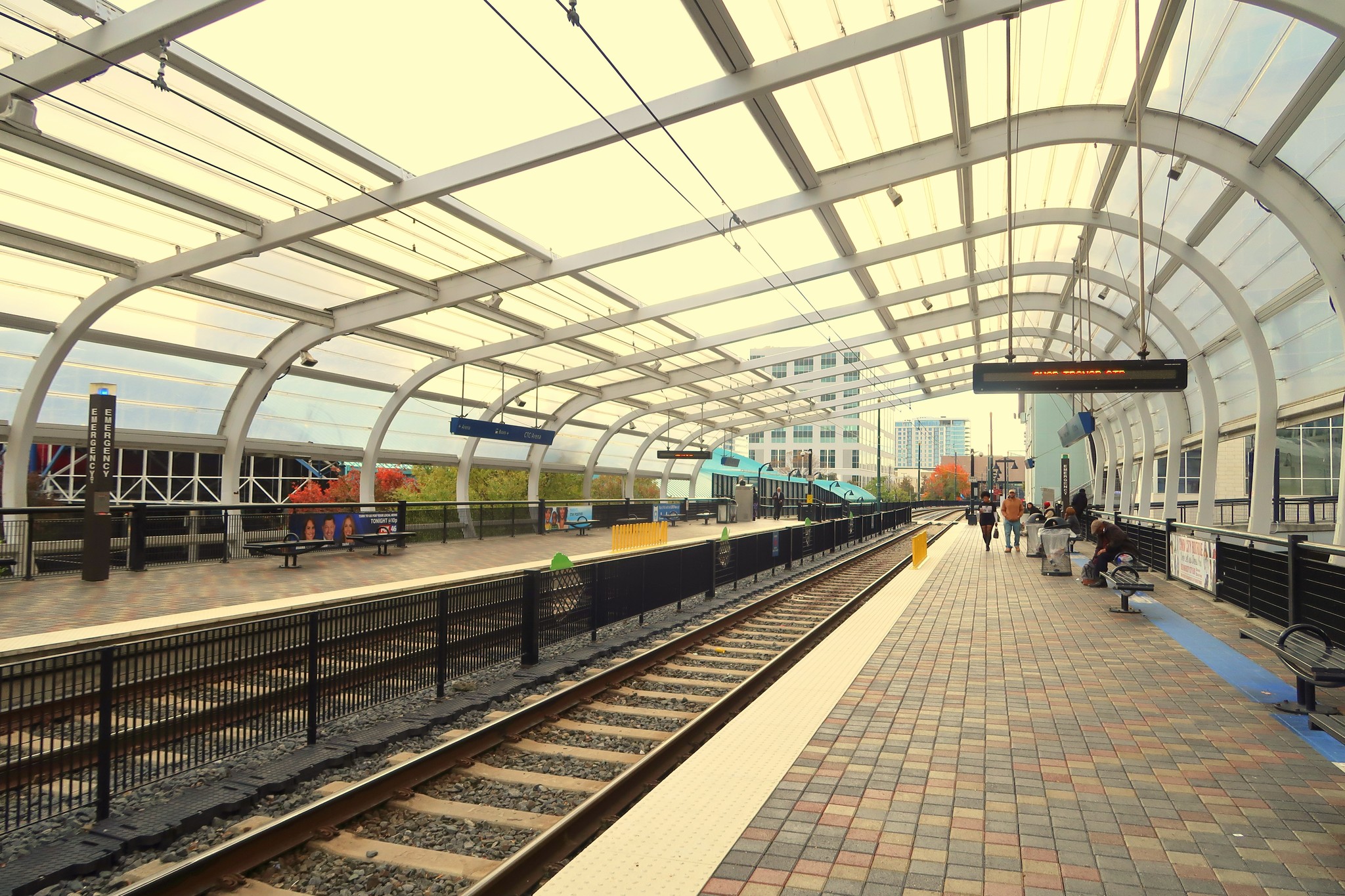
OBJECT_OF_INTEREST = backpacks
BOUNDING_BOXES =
[1080,558,1096,580]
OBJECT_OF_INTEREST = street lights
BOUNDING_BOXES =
[787,468,802,518]
[758,462,774,518]
[844,490,854,516]
[992,457,1018,499]
[830,481,840,503]
[901,473,907,481]
[812,472,824,495]
[934,475,944,497]
[857,496,864,509]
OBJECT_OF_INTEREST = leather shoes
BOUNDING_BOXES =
[1089,579,1107,588]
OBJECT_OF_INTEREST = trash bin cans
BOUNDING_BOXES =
[1020,507,1030,531]
[730,499,738,523]
[1026,513,1046,558]
[1038,517,1072,576]
[717,497,730,523]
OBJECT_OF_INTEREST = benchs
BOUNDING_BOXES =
[243,527,417,568]
[1239,624,1345,744]
[1100,551,1154,614]
[563,510,716,535]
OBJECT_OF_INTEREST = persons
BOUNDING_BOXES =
[339,515,364,545]
[1203,541,1216,593]
[298,515,316,540]
[556,507,569,529]
[1071,488,1087,520]
[1064,507,1081,536]
[1020,502,1056,536]
[1027,503,1042,516]
[319,514,341,547]
[752,486,758,521]
[545,508,553,530]
[977,491,998,551]
[1001,490,1024,552]
[772,487,785,520]
[548,511,559,530]
[1081,520,1138,587]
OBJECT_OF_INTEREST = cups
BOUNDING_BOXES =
[1046,547,1065,561]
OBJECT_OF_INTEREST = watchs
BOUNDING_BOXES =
[1018,516,1020,518]
[1104,549,1105,550]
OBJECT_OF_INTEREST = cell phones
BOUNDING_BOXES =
[980,508,983,513]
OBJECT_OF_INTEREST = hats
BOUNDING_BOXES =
[1044,502,1050,507]
[1009,490,1015,493]
[1091,520,1102,534]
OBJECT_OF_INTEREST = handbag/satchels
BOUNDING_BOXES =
[994,526,998,538]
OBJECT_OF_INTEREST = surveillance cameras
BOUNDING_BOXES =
[979,453,983,457]
[800,452,806,457]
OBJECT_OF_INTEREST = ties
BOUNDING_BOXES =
[778,492,779,498]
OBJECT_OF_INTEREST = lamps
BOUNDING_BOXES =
[1069,346,1078,355]
[886,184,903,207]
[950,382,956,390]
[1098,285,1111,300]
[299,350,318,367]
[484,291,502,311]
[629,421,636,429]
[650,359,662,372]
[922,297,932,311]
[1166,154,1188,181]
[786,415,792,423]
[941,352,948,362]
[514,397,526,407]
[698,436,704,443]
[737,395,744,404]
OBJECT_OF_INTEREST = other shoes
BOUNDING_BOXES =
[986,545,989,551]
[1005,547,1011,552]
[774,517,776,520]
[1015,547,1020,551]
